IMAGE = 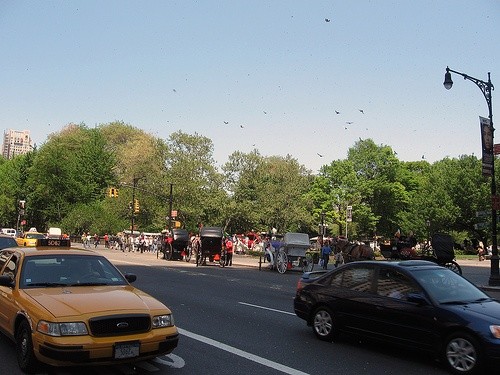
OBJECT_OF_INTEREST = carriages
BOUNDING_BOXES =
[258,232,315,275]
[196,226,226,268]
[160,227,193,262]
[331,231,462,279]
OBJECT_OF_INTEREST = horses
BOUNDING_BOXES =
[333,235,376,268]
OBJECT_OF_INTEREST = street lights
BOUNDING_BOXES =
[442,66,500,290]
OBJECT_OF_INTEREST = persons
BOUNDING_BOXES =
[20,230,272,266]
[321,242,333,270]
[78,262,97,281]
[478,242,485,261]
[387,275,414,300]
[482,124,492,154]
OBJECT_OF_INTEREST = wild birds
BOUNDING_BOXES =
[422,155,424,159]
[224,108,366,158]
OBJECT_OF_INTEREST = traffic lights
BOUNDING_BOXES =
[110,188,115,198]
[135,200,139,213]
[114,189,119,199]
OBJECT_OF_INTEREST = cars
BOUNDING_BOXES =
[0,227,179,372]
[293,259,500,375]
[0,233,19,249]
[16,227,48,247]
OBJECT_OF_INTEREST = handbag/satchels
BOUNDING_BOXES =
[320,259,325,265]
